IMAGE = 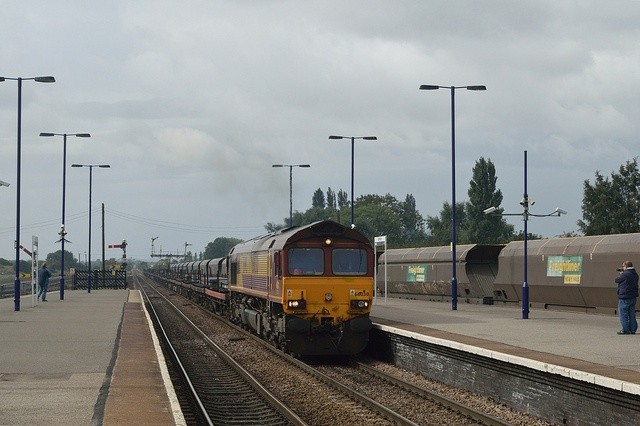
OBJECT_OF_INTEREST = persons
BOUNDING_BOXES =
[38,262,52,301]
[614,261,638,335]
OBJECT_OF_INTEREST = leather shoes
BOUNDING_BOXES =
[617,329,630,334]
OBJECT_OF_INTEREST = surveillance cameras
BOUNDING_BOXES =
[556,207,568,217]
[483,206,498,216]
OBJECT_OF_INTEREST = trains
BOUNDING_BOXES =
[374,231,639,318]
[142,219,376,358]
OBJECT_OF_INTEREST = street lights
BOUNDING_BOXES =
[418,83,487,311]
[272,164,311,227]
[38,132,91,301]
[0,76,56,312]
[70,163,111,292]
[328,134,377,229]
